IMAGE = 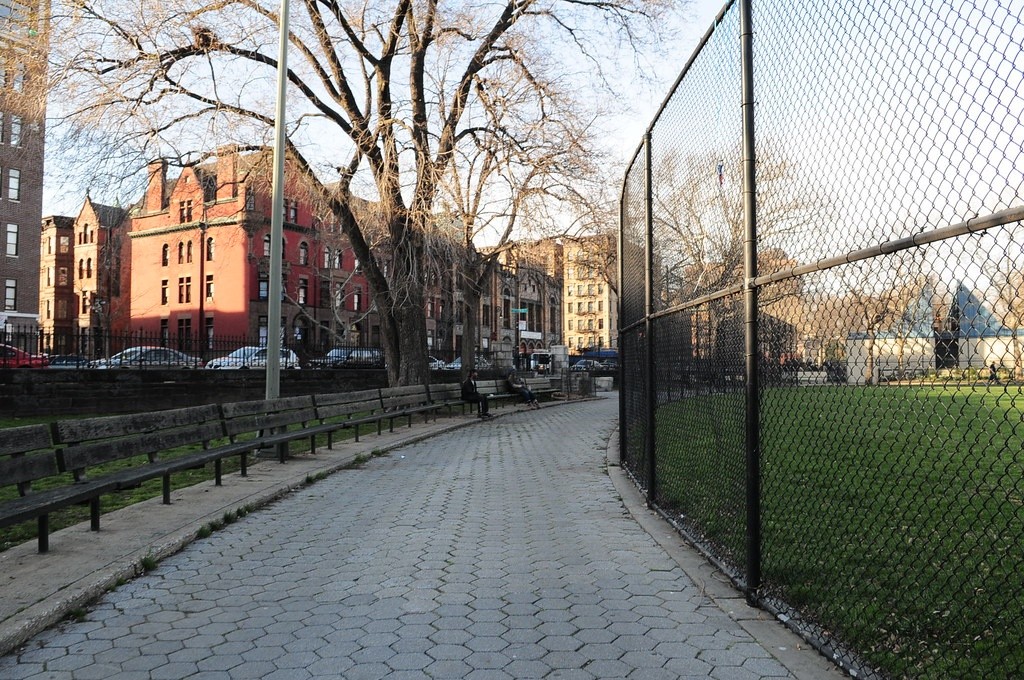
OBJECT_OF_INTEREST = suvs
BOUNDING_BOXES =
[304,347,384,370]
[205,345,301,369]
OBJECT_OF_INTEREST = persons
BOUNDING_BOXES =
[987,361,1000,384]
[508,369,541,409]
[460,368,494,417]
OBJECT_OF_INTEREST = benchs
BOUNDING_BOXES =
[0,377,562,558]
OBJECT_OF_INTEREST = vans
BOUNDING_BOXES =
[530,352,551,374]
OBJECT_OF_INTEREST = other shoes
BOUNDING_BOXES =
[484,413,494,418]
[477,414,489,419]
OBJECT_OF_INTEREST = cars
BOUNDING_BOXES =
[47,354,90,369]
[570,359,603,371]
[90,345,204,370]
[429,355,445,370]
[0,344,48,369]
[599,362,618,371]
[442,356,493,370]
